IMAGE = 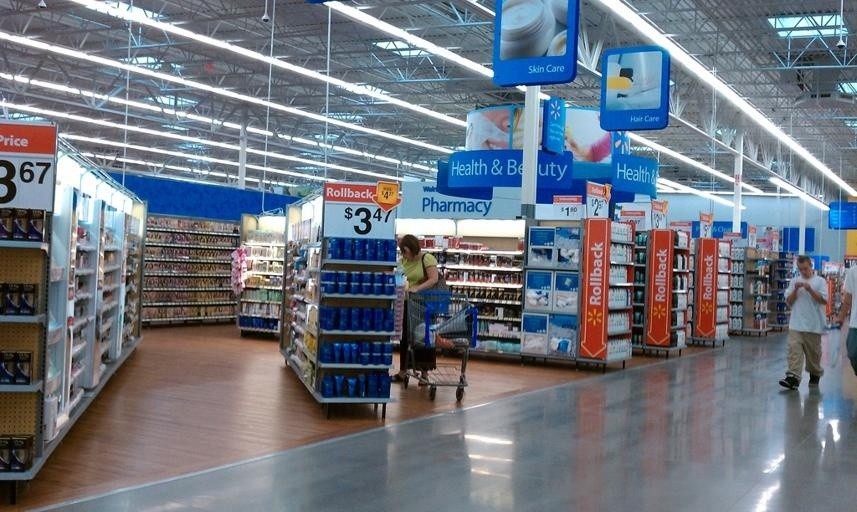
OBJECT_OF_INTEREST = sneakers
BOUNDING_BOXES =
[809,375,819,385]
[779,377,798,389]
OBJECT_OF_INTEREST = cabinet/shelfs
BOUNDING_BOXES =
[238,240,287,341]
[402,233,526,344]
[145,208,239,325]
[285,186,401,407]
[543,215,634,369]
[0,115,141,507]
[638,216,847,359]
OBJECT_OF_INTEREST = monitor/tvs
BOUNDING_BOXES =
[829,202,857,230]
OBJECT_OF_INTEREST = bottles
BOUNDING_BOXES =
[434,252,523,336]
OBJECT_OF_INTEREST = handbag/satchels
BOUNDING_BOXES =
[418,254,447,299]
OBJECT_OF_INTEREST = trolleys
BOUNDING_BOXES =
[400,289,480,404]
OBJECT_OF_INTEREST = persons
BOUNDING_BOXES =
[833,259,857,377]
[565,116,613,162]
[779,256,829,390]
[473,107,524,150]
[391,235,439,386]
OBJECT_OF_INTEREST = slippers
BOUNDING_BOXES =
[419,377,428,386]
[390,374,403,382]
